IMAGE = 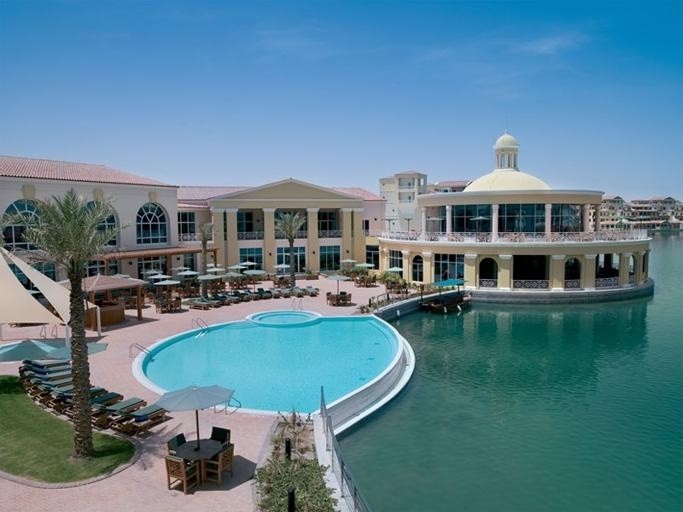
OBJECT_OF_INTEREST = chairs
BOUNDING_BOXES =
[113,276,320,311]
[165,426,234,495]
[336,269,407,293]
[18,359,170,439]
[326,291,352,306]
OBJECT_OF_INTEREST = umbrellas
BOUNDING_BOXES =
[273,264,290,268]
[155,384,235,448]
[141,261,266,301]
[385,266,404,272]
[340,259,357,262]
[356,263,375,267]
